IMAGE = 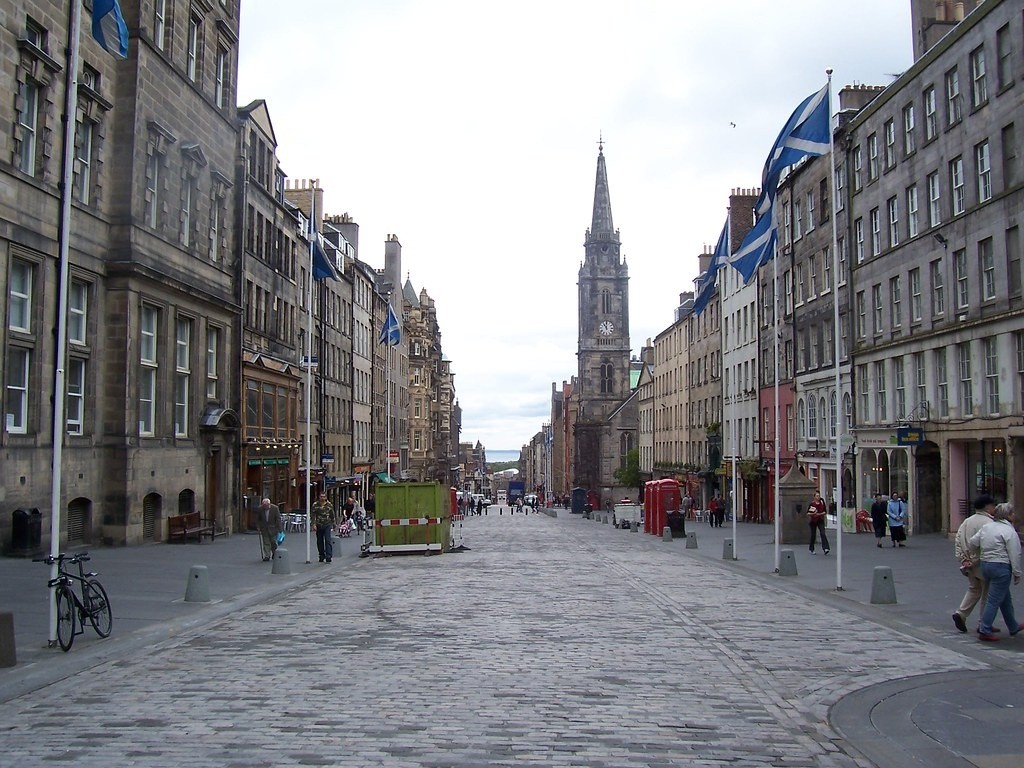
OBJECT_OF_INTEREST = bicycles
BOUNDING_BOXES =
[32,551,114,652]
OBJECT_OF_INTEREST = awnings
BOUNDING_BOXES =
[376,473,397,484]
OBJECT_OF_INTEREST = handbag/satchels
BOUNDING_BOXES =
[277,532,285,545]
[898,500,902,516]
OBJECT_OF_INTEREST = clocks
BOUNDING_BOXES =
[598,320,614,337]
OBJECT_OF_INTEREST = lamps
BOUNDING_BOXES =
[934,233,947,243]
[298,331,306,339]
[256,438,298,451]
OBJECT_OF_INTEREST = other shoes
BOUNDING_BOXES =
[326,558,332,561]
[320,557,324,561]
[979,633,1000,641]
[1009,623,1024,636]
[824,550,829,555]
[263,557,269,560]
[977,625,1001,633]
[899,544,905,546]
[877,543,882,547]
[893,544,896,547]
[952,612,967,633]
[809,550,817,554]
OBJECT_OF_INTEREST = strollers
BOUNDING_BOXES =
[336,516,354,538]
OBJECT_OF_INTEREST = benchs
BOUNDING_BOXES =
[168,511,216,545]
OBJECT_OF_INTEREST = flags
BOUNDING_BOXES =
[692,214,730,316]
[378,302,400,347]
[92,0,129,61]
[308,193,343,284]
[753,83,833,215]
[726,191,779,286]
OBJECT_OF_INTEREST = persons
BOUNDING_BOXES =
[455,496,483,516]
[311,492,335,564]
[339,495,367,537]
[606,499,611,513]
[681,493,725,528]
[886,492,907,547]
[806,492,830,555]
[871,494,888,548]
[952,496,1001,633]
[505,497,541,514]
[970,502,1024,641]
[256,498,283,561]
[558,496,568,510]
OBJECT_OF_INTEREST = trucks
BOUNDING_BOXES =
[468,494,491,509]
[506,481,526,506]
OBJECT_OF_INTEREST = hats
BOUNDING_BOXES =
[355,511,363,515]
[974,494,995,510]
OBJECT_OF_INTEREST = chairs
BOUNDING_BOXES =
[281,513,307,533]
[693,508,726,522]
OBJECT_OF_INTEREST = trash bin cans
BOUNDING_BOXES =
[12,508,42,549]
[666,510,686,538]
